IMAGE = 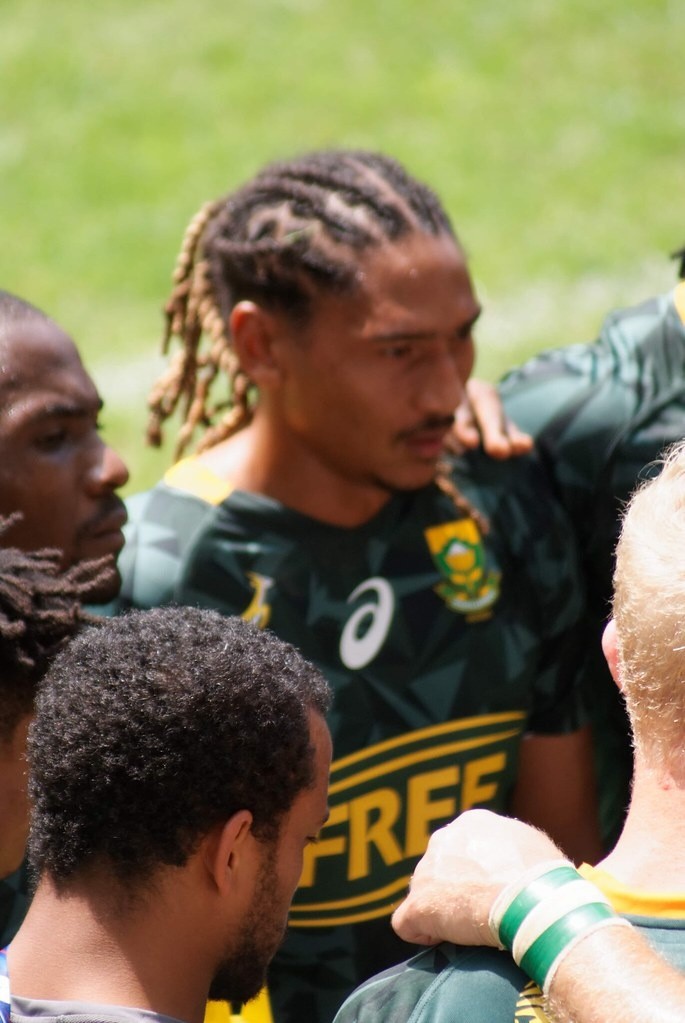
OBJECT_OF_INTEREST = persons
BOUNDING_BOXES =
[333,437,685,1023]
[0,614,685,1023]
[0,147,685,1023]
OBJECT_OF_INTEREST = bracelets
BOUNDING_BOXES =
[488,860,635,998]
